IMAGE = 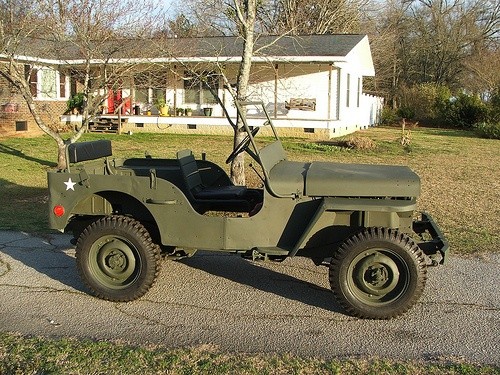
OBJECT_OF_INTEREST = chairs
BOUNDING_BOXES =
[175,149,250,212]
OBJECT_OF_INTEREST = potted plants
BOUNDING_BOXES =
[156,89,170,117]
[202,97,213,117]
[175,107,184,117]
[186,108,193,117]
[146,104,152,116]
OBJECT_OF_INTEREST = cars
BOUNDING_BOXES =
[46,100,449,319]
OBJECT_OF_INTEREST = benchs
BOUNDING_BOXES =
[65,138,136,177]
